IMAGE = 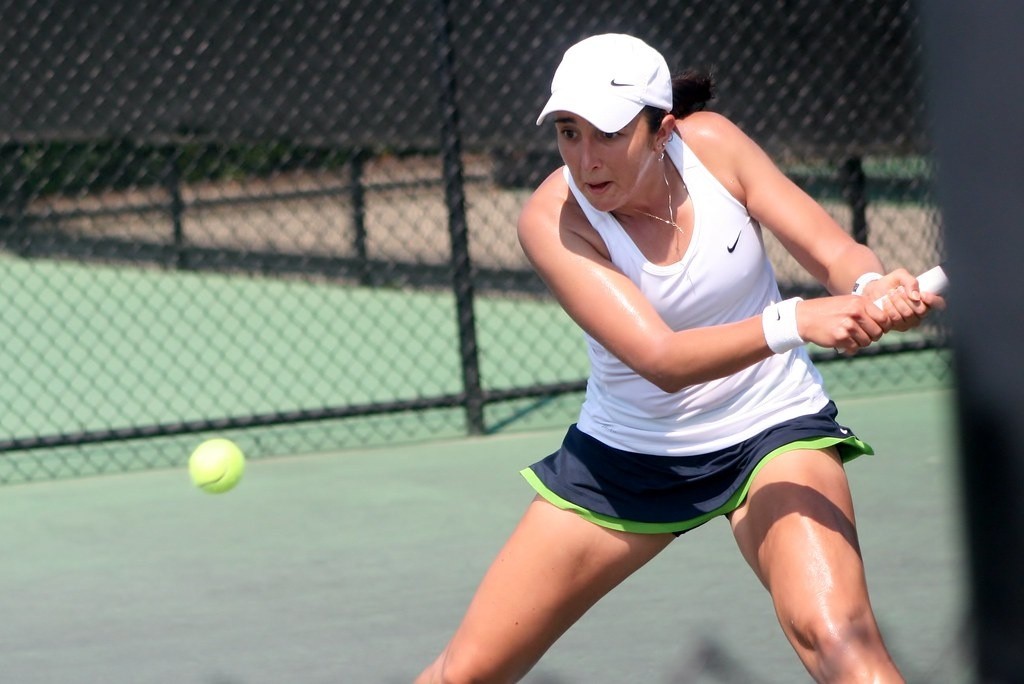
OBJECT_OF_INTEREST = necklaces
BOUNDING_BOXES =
[633,171,687,234]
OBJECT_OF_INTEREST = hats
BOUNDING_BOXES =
[537,34,673,134]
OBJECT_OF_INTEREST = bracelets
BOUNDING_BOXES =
[851,271,883,296]
[762,297,809,354]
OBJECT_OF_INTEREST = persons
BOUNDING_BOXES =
[413,32,947,684]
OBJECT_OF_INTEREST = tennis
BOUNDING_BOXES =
[187,438,249,494]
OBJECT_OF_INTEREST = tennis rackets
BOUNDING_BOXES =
[872,262,950,319]
